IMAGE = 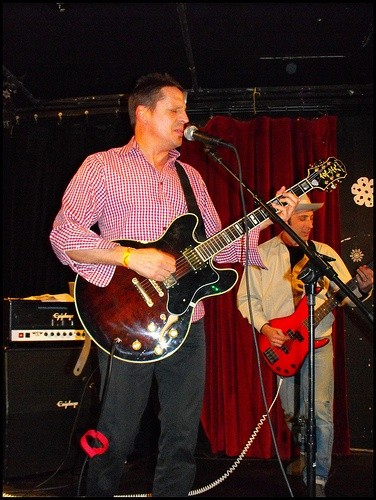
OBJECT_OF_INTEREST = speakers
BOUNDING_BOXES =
[0,344,101,481]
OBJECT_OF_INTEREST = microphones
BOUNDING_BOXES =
[183,125,223,149]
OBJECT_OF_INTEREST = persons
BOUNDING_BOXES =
[50,71,304,500]
[237,186,376,500]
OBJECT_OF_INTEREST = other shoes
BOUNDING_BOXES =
[315,483,326,497]
[286,454,307,475]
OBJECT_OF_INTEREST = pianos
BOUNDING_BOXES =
[2,295,91,344]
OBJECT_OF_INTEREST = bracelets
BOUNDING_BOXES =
[120,247,135,269]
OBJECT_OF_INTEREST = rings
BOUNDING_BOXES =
[363,279,367,282]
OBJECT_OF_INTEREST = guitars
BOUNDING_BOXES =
[257,260,375,378]
[74,154,348,366]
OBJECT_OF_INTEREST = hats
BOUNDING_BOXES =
[295,193,324,210]
